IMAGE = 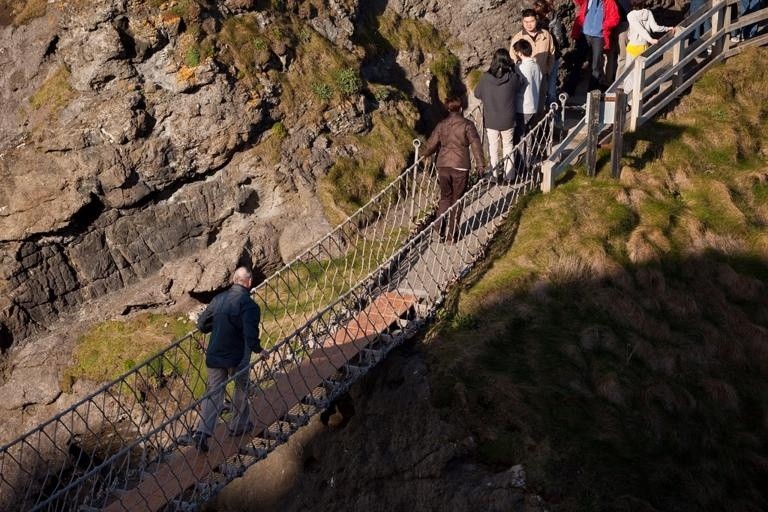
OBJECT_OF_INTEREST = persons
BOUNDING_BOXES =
[689,0,713,53]
[193,267,269,453]
[727,0,744,43]
[474,1,675,186]
[421,96,487,245]
[739,0,761,42]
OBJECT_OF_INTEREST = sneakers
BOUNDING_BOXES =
[194,432,210,453]
[229,420,254,436]
